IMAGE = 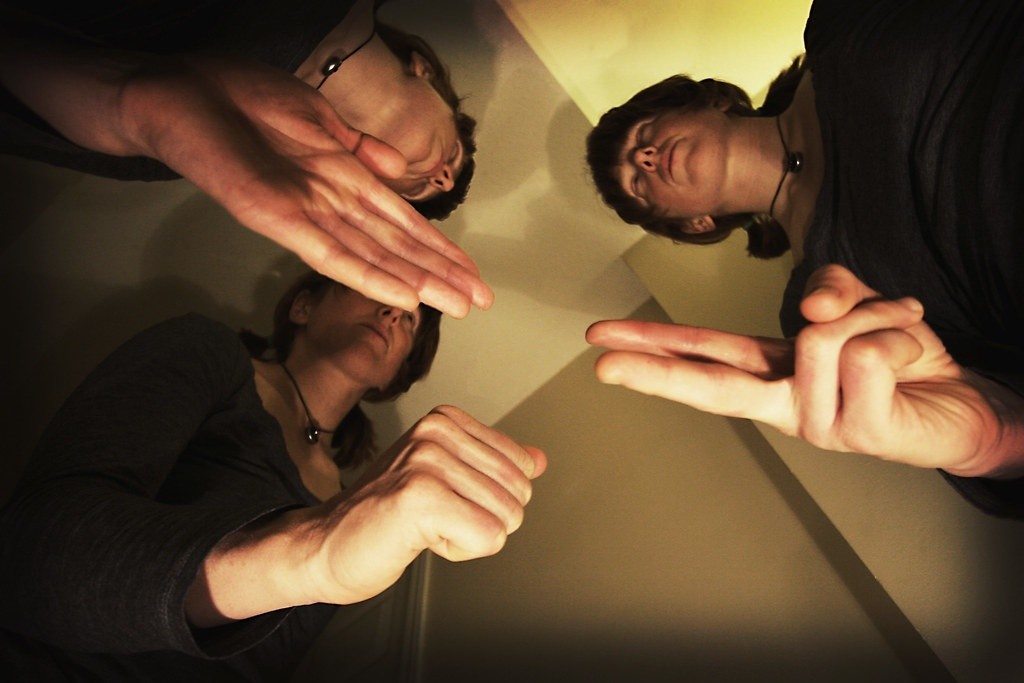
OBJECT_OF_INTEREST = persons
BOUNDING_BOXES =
[586,0,1024,518]
[0,272,547,683]
[0,0,495,319]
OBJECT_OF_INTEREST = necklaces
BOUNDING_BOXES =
[770,117,802,218]
[319,32,377,91]
[279,362,335,444]
